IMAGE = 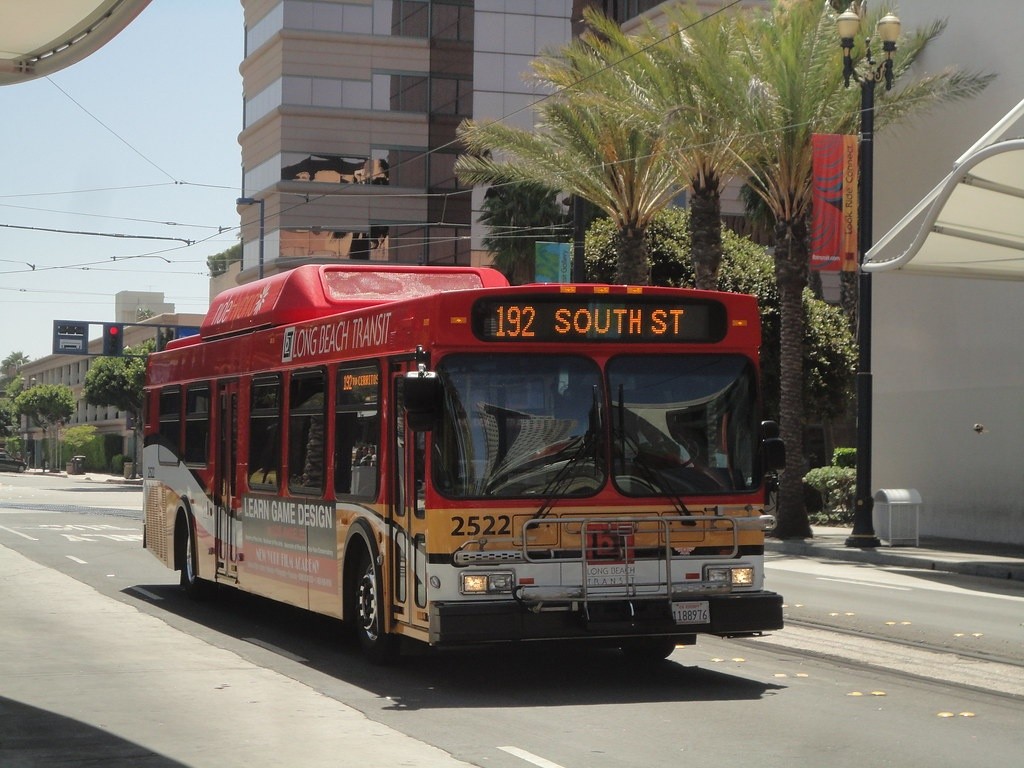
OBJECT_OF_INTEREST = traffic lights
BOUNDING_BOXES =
[103,322,124,355]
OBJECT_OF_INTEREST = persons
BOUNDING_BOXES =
[355,445,376,467]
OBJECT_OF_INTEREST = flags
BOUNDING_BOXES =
[534,243,572,284]
[810,132,857,271]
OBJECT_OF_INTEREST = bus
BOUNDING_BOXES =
[136,263,785,658]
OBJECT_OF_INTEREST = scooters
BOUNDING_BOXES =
[762,467,779,519]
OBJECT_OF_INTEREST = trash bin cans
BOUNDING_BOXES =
[873,488,923,547]
[73,456,86,474]
[124,462,138,479]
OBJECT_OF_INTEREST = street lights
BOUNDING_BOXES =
[835,5,903,548]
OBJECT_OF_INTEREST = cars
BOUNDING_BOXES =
[0,447,27,474]
